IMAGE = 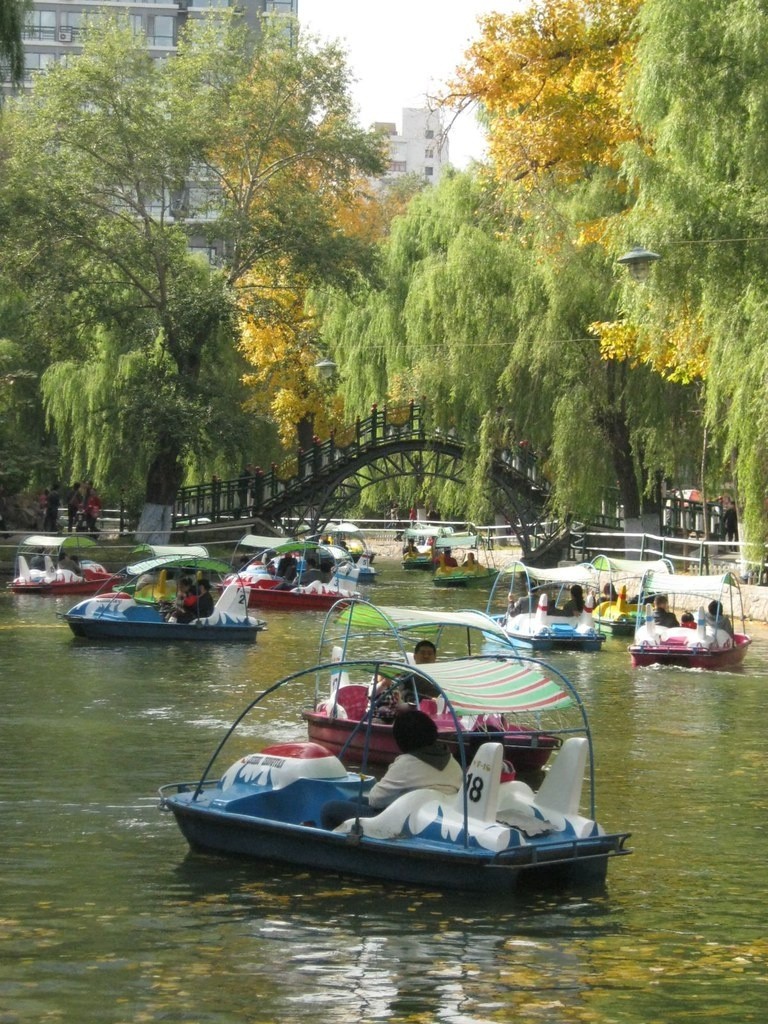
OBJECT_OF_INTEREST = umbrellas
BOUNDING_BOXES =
[665,490,709,506]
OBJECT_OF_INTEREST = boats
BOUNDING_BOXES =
[484,560,606,652]
[588,554,675,637]
[55,555,267,644]
[628,569,753,670]
[401,523,498,589]
[126,522,379,609]
[157,598,631,902]
[7,536,123,595]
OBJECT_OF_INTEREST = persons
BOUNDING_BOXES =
[508,580,541,617]
[0,480,101,539]
[681,610,697,629]
[402,536,479,567]
[136,566,214,625]
[653,595,680,628]
[297,413,316,465]
[495,405,516,466]
[239,534,349,591]
[400,640,442,703]
[466,725,516,783]
[666,489,738,576]
[562,584,584,616]
[597,583,618,605]
[320,710,463,831]
[29,547,88,580]
[705,600,733,636]
[366,662,415,725]
[391,503,431,542]
[630,590,655,605]
